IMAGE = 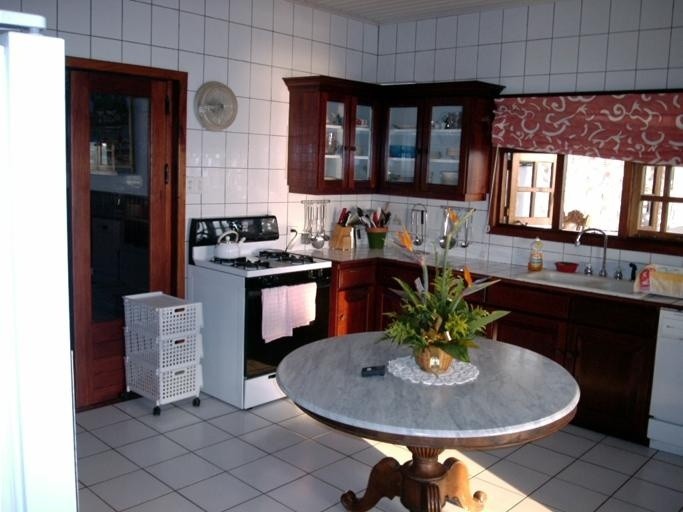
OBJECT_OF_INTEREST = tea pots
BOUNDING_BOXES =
[214,230,247,260]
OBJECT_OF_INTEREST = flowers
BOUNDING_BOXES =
[374,208,510,363]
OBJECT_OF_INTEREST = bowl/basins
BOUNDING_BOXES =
[555,261,578,273]
[390,145,416,158]
[438,169,459,184]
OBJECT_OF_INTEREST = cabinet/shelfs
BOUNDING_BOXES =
[119,288,204,416]
[375,257,498,338]
[484,274,661,447]
[283,74,378,197]
[327,259,375,336]
[378,81,506,202]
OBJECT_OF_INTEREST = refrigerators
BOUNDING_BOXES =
[1,10,82,512]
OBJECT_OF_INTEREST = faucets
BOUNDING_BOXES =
[575,227,608,278]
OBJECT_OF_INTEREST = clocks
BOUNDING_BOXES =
[195,81,239,134]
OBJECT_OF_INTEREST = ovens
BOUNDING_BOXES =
[245,267,331,411]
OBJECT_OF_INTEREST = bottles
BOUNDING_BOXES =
[527,234,546,271]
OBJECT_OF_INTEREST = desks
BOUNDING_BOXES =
[276,328,580,512]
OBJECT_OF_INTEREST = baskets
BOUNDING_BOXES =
[124,326,203,368]
[125,356,203,404]
[122,291,203,335]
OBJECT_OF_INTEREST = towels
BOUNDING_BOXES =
[261,285,294,344]
[287,281,317,329]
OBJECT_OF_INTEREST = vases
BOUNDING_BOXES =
[414,345,453,374]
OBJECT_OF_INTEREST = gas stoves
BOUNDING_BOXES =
[209,249,329,272]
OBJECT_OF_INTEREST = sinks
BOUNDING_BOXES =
[527,271,615,291]
